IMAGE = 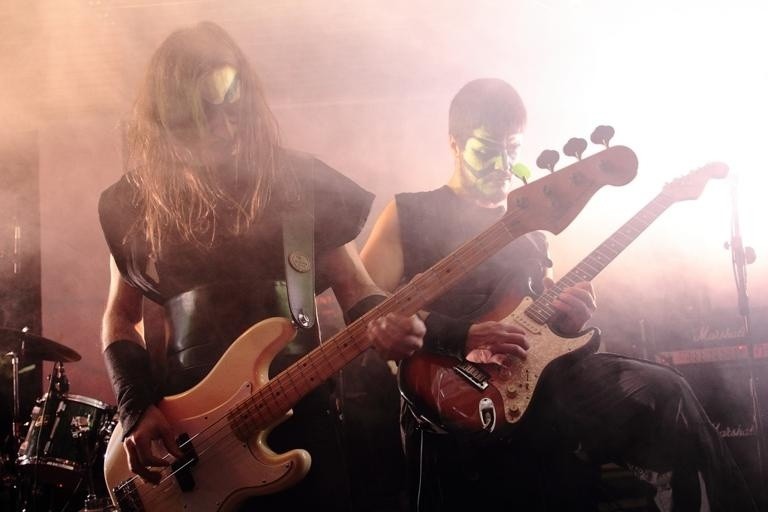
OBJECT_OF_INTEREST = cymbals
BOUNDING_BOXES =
[1,328,82,362]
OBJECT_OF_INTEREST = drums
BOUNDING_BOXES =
[16,392,119,484]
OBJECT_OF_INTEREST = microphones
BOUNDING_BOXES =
[58,362,69,393]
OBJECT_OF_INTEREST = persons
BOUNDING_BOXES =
[97,21,429,509]
[341,78,758,509]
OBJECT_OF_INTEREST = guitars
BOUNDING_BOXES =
[398,165,728,446]
[104,126,638,510]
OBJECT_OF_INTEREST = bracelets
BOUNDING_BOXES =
[422,309,473,362]
